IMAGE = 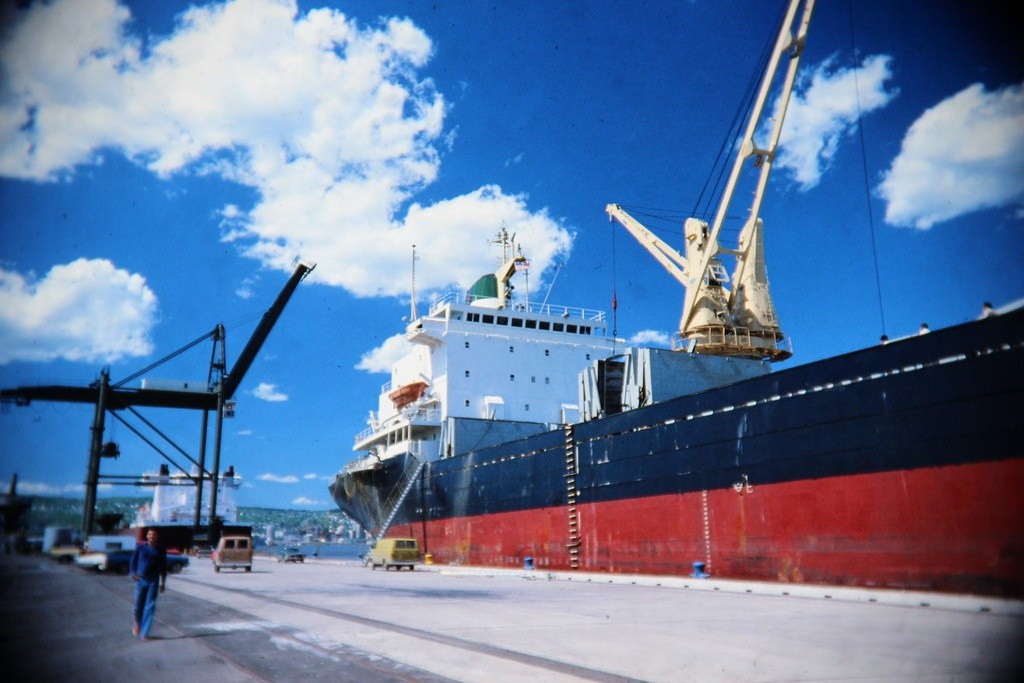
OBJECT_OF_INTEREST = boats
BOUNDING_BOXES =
[328,0,1023,604]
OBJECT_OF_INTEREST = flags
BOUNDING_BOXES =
[515,260,528,267]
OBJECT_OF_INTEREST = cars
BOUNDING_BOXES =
[275,547,305,563]
[75,550,190,574]
[48,546,83,565]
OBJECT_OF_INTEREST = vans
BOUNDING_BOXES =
[366,539,419,571]
[211,536,256,573]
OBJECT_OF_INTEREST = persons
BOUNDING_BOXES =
[130,530,168,642]
[919,322,931,335]
[981,301,997,319]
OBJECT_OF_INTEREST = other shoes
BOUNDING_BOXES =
[132,625,140,636]
[141,638,147,642]
